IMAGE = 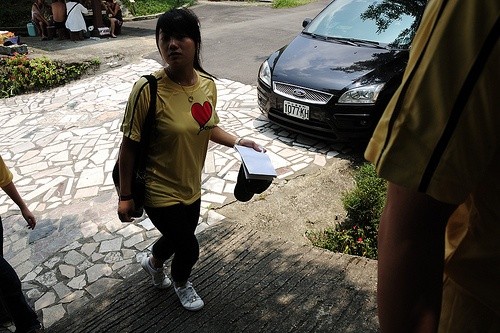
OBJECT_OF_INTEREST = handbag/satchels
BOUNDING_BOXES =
[112,157,146,218]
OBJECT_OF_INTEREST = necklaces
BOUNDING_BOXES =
[177,80,195,102]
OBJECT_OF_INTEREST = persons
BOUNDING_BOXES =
[363,0,500,333]
[117,8,267,311]
[0,156,39,333]
[31,0,123,41]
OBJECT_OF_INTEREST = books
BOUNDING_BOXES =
[235,146,277,180]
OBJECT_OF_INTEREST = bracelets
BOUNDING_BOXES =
[119,194,132,201]
[235,138,242,144]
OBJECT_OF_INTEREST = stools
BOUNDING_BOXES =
[69,31,83,40]
[55,27,65,39]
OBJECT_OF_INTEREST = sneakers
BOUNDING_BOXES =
[141,257,171,289]
[174,281,204,310]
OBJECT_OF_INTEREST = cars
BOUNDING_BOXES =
[257,0,430,147]
